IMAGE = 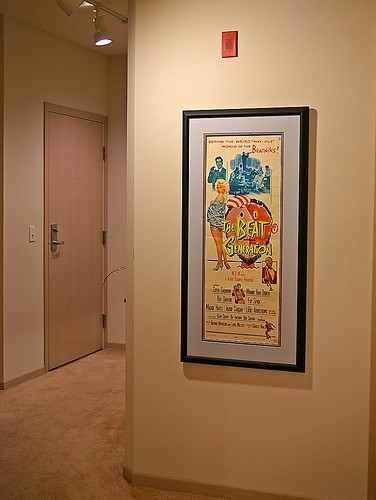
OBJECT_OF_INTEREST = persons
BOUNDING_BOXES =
[207,178,231,270]
[206,155,227,186]
[262,256,276,283]
[242,151,250,171]
[232,284,244,304]
[263,320,275,340]
[230,161,271,194]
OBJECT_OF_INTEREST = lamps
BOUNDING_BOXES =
[93,3,112,47]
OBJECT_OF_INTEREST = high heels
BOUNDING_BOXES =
[213,263,223,271]
[225,263,231,269]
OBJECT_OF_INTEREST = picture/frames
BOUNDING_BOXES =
[179,106,311,372]
